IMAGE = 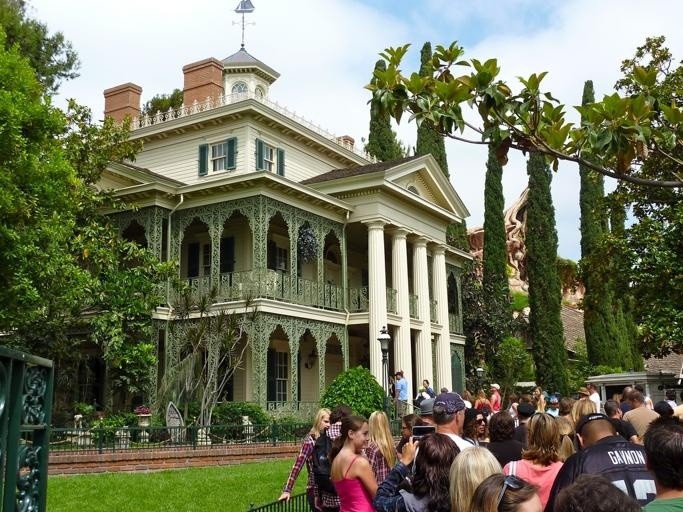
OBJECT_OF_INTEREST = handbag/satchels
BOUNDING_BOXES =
[416,396,426,406]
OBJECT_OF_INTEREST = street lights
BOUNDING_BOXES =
[475,366,485,389]
[376,323,392,437]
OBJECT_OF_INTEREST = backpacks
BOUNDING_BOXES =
[312,429,337,496]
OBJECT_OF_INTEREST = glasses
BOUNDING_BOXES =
[496,475,524,507]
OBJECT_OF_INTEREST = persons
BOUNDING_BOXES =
[277,372,683,512]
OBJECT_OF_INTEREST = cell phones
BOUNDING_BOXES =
[412,426,436,438]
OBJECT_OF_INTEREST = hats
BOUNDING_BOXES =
[433,392,465,416]
[395,372,402,376]
[517,404,534,417]
[490,384,500,390]
[576,413,608,432]
[578,387,591,397]
[419,398,435,415]
